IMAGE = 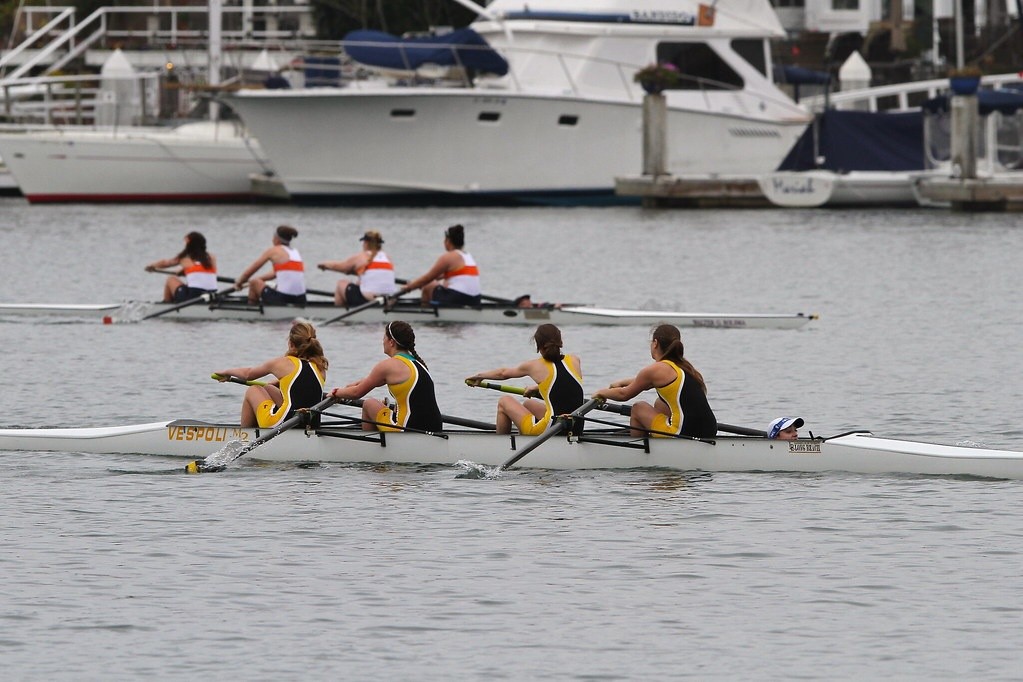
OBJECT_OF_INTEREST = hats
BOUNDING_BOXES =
[768,415,804,439]
[358,234,383,245]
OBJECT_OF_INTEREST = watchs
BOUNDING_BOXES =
[331,387,339,396]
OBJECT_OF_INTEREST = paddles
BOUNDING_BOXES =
[145,267,334,297]
[104,282,249,324]
[499,396,605,470]
[211,373,497,431]
[318,265,513,303]
[466,379,767,436]
[316,288,408,327]
[185,396,339,473]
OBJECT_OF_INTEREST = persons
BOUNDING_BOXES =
[325,321,443,431]
[590,324,717,438]
[512,295,533,308]
[213,322,328,429]
[767,417,805,439]
[233,225,306,305]
[144,231,218,303]
[465,324,585,436]
[317,229,396,306]
[400,225,481,304]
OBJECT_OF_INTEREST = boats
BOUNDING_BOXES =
[1,419,1023,483]
[0,72,282,205]
[212,0,813,196]
[755,105,1005,206]
[0,159,20,198]
[0,296,819,329]
